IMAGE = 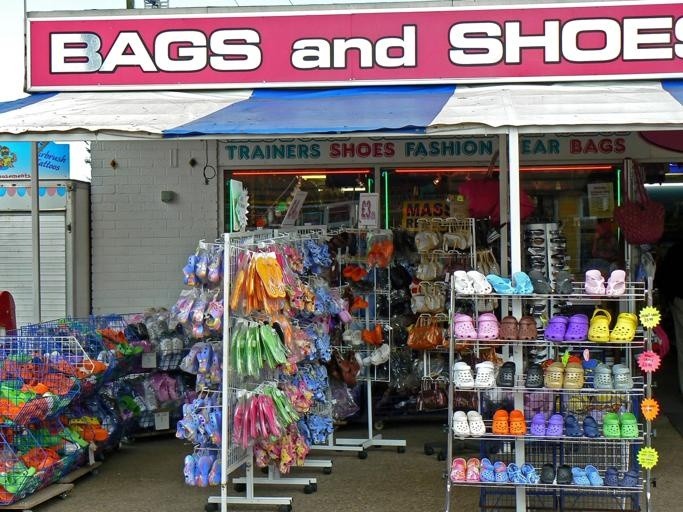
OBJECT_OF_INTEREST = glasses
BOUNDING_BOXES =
[526,229,568,284]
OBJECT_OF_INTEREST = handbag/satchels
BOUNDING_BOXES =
[458,150,535,226]
[613,203,666,245]
[453,391,478,409]
[413,216,473,412]
[390,265,412,289]
[446,250,500,275]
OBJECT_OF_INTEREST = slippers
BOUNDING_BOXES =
[453,356,634,391]
[0,313,149,504]
[453,409,639,439]
[334,349,361,386]
[452,457,639,488]
[454,309,638,343]
[452,271,640,296]
[364,343,390,367]
[149,307,185,414]
[343,331,361,346]
[362,325,382,344]
[175,255,221,487]
[352,297,368,311]
[230,238,332,474]
[342,266,367,282]
[367,239,393,268]
